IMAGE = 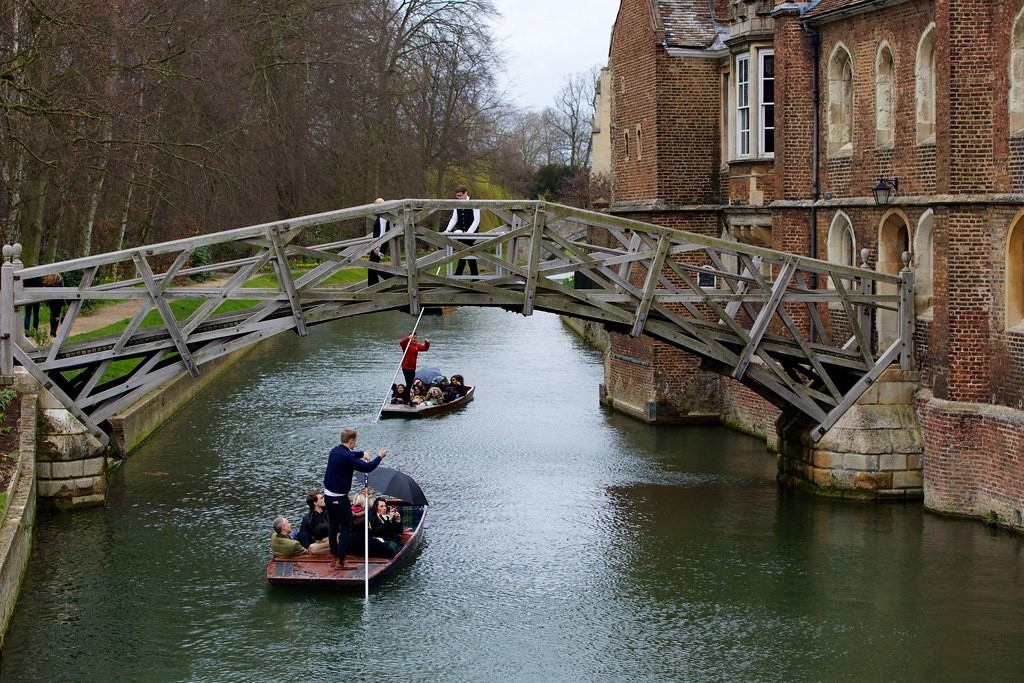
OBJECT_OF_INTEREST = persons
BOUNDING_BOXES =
[271,489,402,560]
[367,198,395,287]
[23,264,44,332]
[391,374,468,407]
[43,273,66,337]
[443,185,480,282]
[323,428,388,570]
[399,332,430,405]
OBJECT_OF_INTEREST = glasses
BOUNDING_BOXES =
[456,193,465,197]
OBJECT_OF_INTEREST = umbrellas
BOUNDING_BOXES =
[415,366,442,384]
[354,468,430,507]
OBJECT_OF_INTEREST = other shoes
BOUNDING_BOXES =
[405,401,418,408]
[330,557,357,570]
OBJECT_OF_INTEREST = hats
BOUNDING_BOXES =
[434,376,444,384]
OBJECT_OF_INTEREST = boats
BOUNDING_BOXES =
[381,385,476,419]
[402,305,455,315]
[267,500,427,594]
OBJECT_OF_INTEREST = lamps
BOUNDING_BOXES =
[871,175,898,208]
